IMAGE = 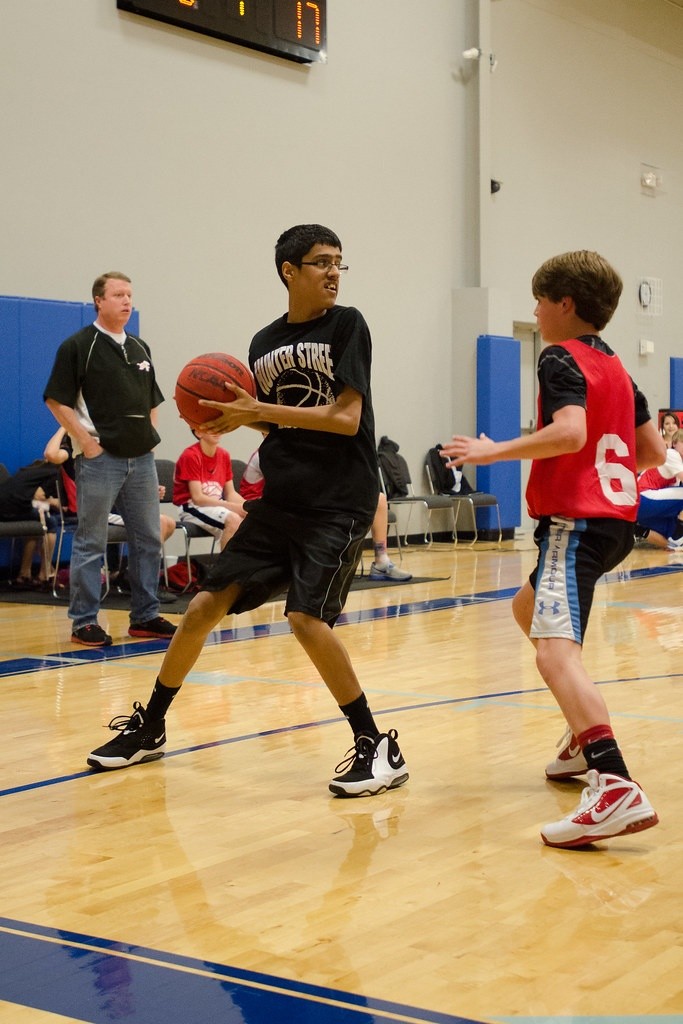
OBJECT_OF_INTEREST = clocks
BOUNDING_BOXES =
[639,282,652,308]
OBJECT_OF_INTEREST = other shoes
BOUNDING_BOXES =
[156,591,177,603]
[10,576,50,593]
[114,569,132,592]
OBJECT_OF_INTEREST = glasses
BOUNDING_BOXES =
[302,259,349,274]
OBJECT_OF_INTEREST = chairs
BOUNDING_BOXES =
[51,453,458,604]
[0,462,50,596]
[425,448,503,550]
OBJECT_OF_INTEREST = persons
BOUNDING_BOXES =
[85,224,408,798]
[638,413,683,564]
[0,423,273,592]
[370,492,412,583]
[44,271,185,645]
[439,248,665,847]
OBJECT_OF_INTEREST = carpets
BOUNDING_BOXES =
[0,569,452,615]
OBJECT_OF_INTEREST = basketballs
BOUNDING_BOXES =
[174,352,258,435]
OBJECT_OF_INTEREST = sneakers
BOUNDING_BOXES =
[545,724,588,780]
[128,616,177,638]
[369,562,412,581]
[667,536,683,550]
[541,770,659,847]
[329,729,409,797]
[87,701,167,770]
[72,625,112,646]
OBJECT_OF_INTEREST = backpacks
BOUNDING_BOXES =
[427,444,472,495]
[160,562,202,595]
